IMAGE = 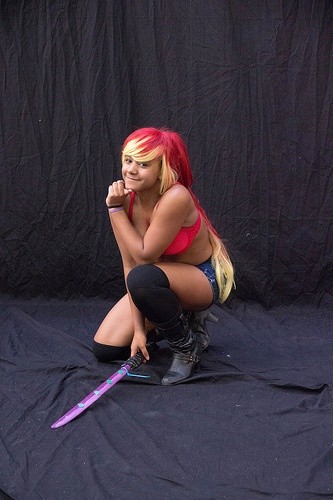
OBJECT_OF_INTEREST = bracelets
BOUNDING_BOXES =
[106,204,124,215]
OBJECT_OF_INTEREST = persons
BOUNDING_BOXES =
[91,126,235,388]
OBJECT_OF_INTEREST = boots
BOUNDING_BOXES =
[149,304,203,386]
[179,309,219,355]
